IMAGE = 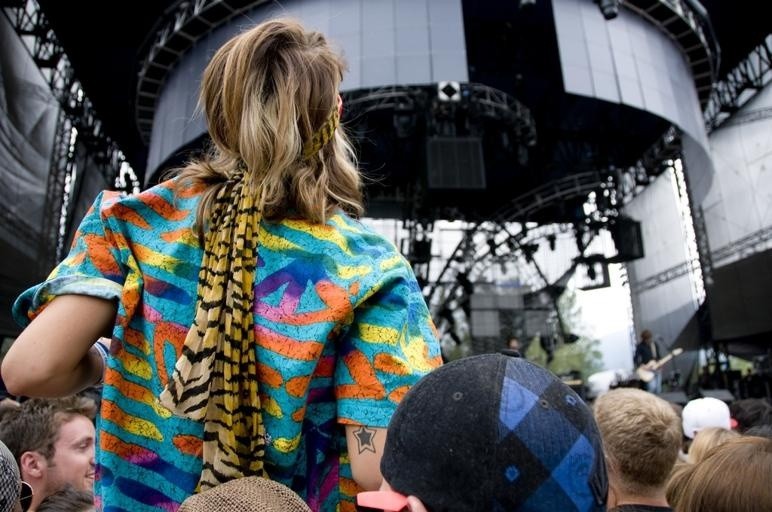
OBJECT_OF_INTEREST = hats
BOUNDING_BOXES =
[380,349,609,512]
[681,397,738,439]
[0,439,22,512]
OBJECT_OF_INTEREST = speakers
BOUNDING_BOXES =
[611,219,644,261]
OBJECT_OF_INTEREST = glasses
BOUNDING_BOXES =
[18,481,33,512]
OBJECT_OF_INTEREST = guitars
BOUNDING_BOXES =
[636,347,683,383]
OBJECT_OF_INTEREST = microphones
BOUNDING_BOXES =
[655,332,664,342]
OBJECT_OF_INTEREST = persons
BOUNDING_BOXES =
[0,19,447,512]
[0,328,771,512]
